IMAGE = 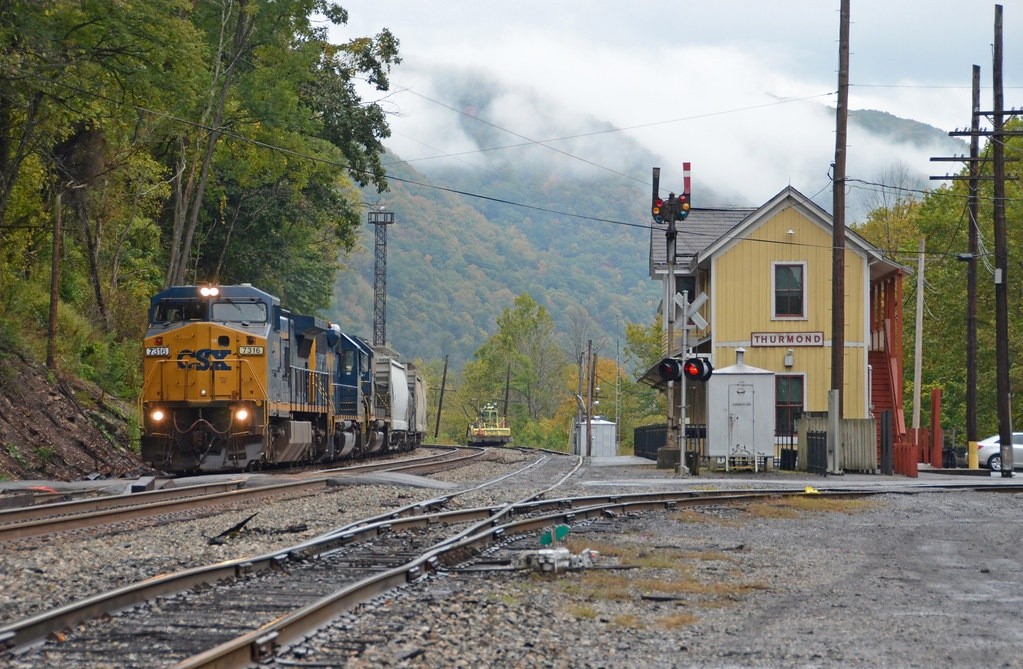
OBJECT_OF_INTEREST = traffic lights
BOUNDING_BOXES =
[684,359,704,380]
[658,357,682,382]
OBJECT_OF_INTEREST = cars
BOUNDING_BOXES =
[964,431,1023,473]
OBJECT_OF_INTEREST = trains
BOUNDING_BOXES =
[139,283,428,478]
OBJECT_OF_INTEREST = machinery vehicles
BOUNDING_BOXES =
[465,404,512,448]
